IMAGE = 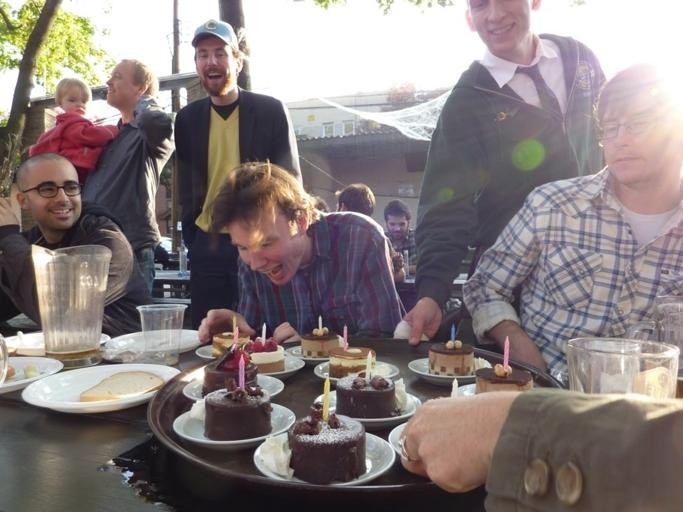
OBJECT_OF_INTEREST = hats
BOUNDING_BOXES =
[192,20,238,51]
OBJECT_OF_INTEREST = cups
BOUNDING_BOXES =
[563,337,679,400]
[134,301,187,367]
[30,244,111,370]
[628,295,682,382]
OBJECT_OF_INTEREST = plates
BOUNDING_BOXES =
[314,360,400,385]
[172,401,297,451]
[285,345,377,364]
[448,382,477,397]
[183,373,286,401]
[203,351,306,380]
[253,429,396,487]
[314,389,423,432]
[408,356,494,386]
[0,329,210,415]
[194,345,285,362]
[386,422,408,457]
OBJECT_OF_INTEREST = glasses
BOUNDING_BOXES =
[595,116,652,140]
[22,180,82,198]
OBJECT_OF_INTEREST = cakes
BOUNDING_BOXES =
[211,333,250,359]
[337,369,399,418]
[329,347,375,378]
[202,348,258,399]
[204,379,273,441]
[429,341,475,376]
[474,364,534,394]
[301,327,339,359]
[241,338,284,373]
[287,404,366,484]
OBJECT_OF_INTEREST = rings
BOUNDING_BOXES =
[399,435,420,463]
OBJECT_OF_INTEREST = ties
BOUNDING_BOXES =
[515,64,563,124]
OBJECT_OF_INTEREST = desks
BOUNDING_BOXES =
[0,263,682,510]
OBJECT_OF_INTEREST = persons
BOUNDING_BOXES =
[399,389,683,512]
[384,200,417,274]
[174,19,303,329]
[30,79,120,185]
[462,65,683,398]
[403,0,607,355]
[82,59,176,295]
[310,196,329,213]
[198,158,405,345]
[0,153,153,339]
[336,184,375,216]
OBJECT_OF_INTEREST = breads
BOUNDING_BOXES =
[79,371,163,402]
